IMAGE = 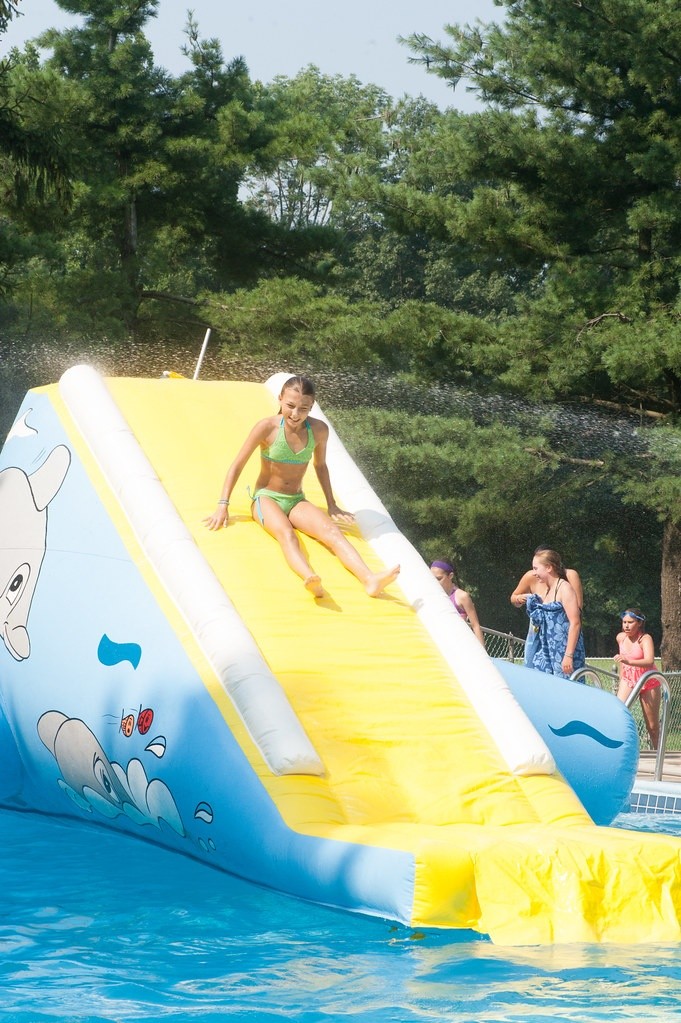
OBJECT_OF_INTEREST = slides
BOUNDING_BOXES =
[0,365,681,948]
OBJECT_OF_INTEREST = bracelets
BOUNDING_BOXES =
[217,499,230,505]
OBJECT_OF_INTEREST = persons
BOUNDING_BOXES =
[532,549,585,687]
[203,375,401,598]
[612,607,663,751]
[429,556,484,652]
[509,545,583,667]
